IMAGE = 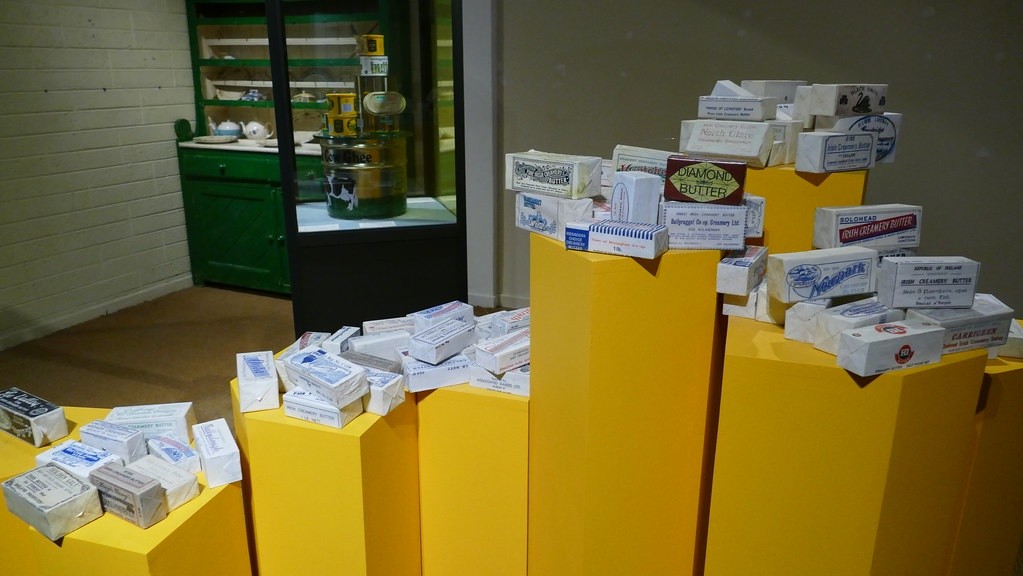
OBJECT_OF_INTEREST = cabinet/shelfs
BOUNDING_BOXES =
[173,0,400,294]
